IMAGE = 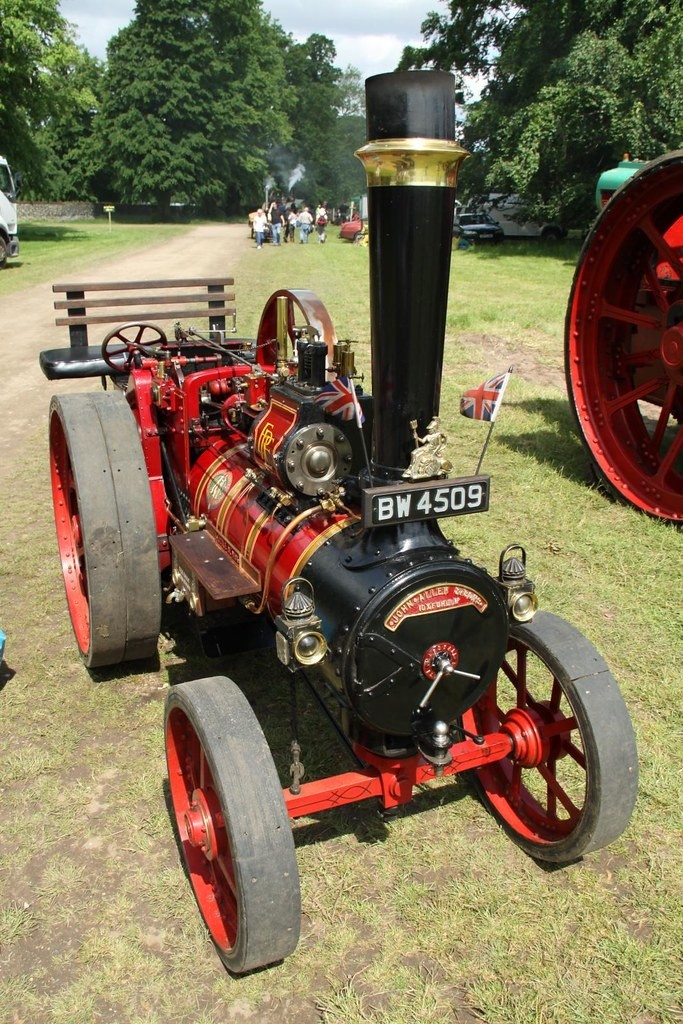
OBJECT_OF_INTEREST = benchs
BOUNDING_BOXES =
[38,276,257,381]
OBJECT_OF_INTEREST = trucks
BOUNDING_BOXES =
[1,157,22,269]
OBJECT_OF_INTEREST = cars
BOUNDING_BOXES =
[452,213,505,245]
[338,219,364,243]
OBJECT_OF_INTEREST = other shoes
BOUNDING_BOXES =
[283,237,287,243]
[257,246,261,249]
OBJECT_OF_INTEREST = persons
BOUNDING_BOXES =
[402,417,441,478]
[336,201,355,226]
[253,194,327,250]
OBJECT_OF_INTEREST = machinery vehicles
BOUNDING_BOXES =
[41,69,640,974]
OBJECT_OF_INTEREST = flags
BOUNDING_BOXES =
[460,373,507,421]
[315,376,365,423]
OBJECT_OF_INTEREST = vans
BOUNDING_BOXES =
[453,193,569,244]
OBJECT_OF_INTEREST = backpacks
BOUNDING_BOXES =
[284,207,290,220]
[317,213,327,226]
